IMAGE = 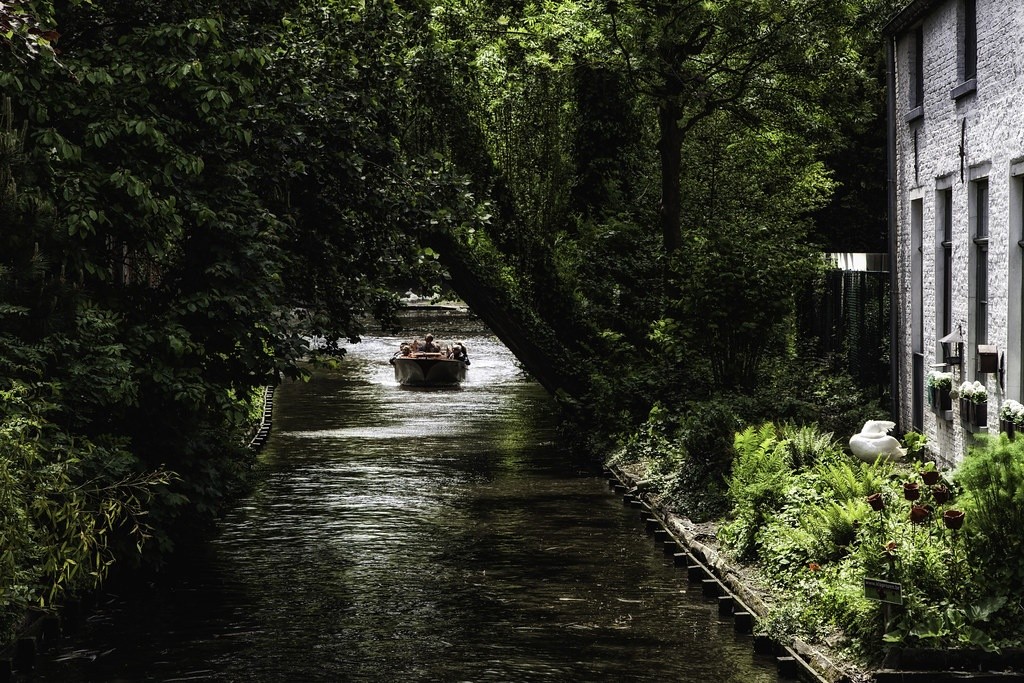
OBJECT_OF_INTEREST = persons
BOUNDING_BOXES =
[399,331,465,361]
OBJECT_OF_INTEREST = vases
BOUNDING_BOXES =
[927,385,952,410]
[959,396,987,427]
[998,417,1024,442]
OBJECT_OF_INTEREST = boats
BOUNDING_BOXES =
[389,339,471,390]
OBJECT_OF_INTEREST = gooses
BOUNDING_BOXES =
[848,419,908,465]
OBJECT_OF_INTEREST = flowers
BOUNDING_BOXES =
[925,370,952,390]
[1001,398,1024,423]
[958,380,987,402]
